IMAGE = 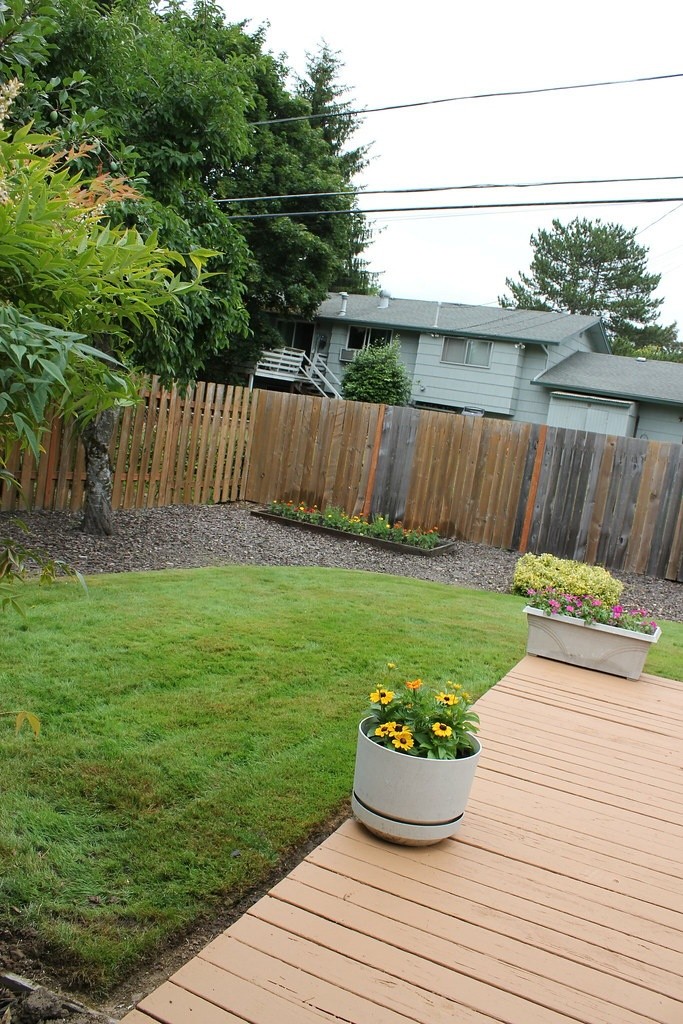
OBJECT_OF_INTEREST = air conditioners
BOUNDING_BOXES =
[338,348,359,362]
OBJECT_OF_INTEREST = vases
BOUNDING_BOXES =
[350,715,483,848]
[522,605,663,682]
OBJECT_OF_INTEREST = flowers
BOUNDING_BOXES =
[369,663,480,759]
[527,584,658,636]
